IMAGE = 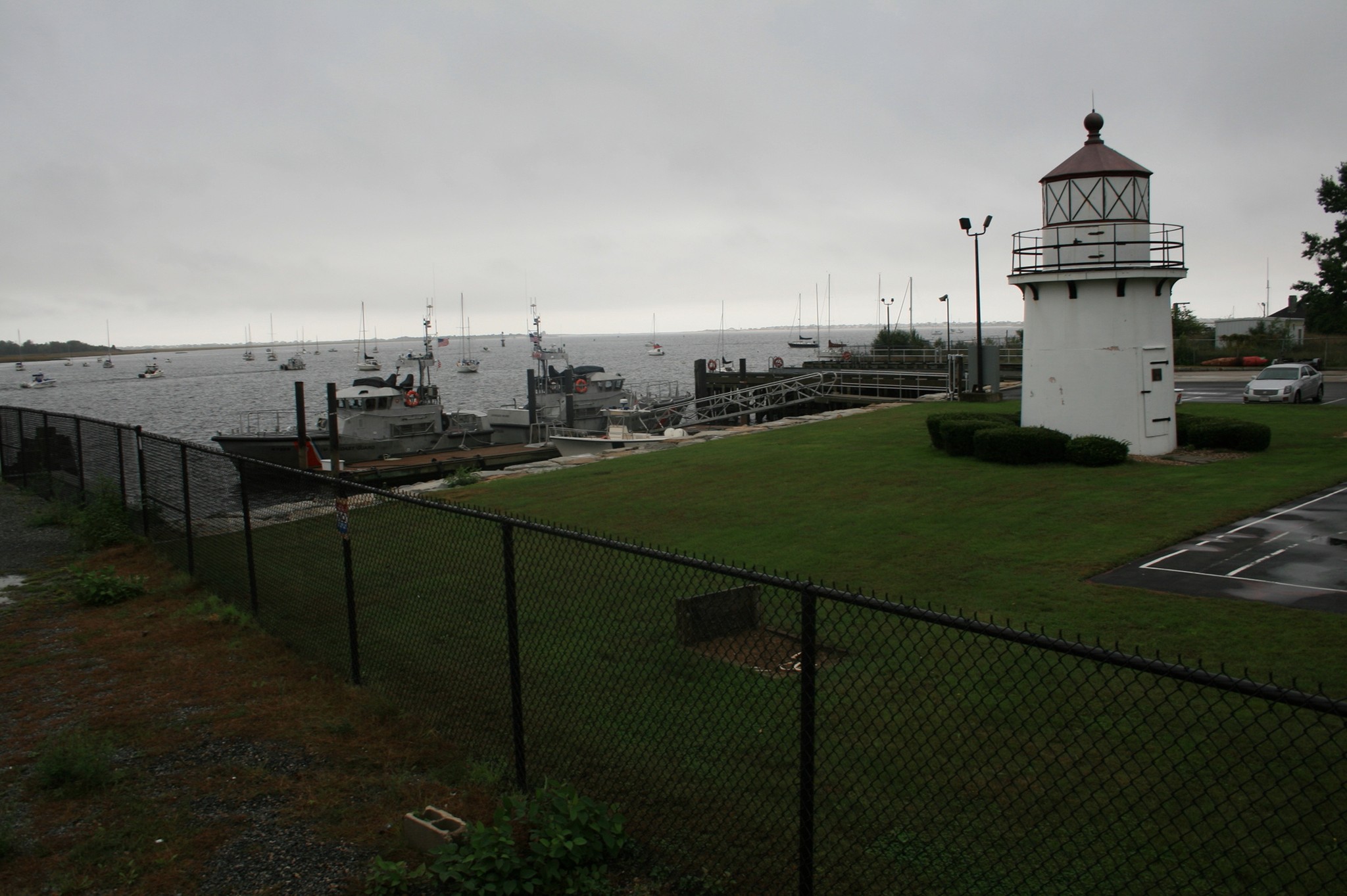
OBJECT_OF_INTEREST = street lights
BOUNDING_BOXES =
[881,297,894,361]
[960,216,993,392]
[939,294,951,354]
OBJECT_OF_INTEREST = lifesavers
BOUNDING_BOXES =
[708,361,717,370]
[774,357,783,367]
[406,390,420,406]
[575,379,588,393]
[843,351,851,360]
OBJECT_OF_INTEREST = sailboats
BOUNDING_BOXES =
[787,294,820,347]
[102,319,115,368]
[356,302,382,371]
[827,274,847,347]
[15,330,26,371]
[242,313,380,361]
[457,292,480,372]
[648,313,665,355]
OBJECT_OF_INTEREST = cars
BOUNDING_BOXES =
[1243,363,1325,404]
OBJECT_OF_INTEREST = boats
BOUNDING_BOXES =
[487,304,695,446]
[166,360,172,363]
[645,342,656,347]
[138,364,164,378]
[83,363,90,367]
[65,362,74,366]
[211,319,563,488]
[98,359,103,363]
[280,351,308,370]
[28,373,56,388]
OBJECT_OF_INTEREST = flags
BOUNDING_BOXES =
[529,333,538,342]
[437,338,449,347]
[438,360,441,368]
